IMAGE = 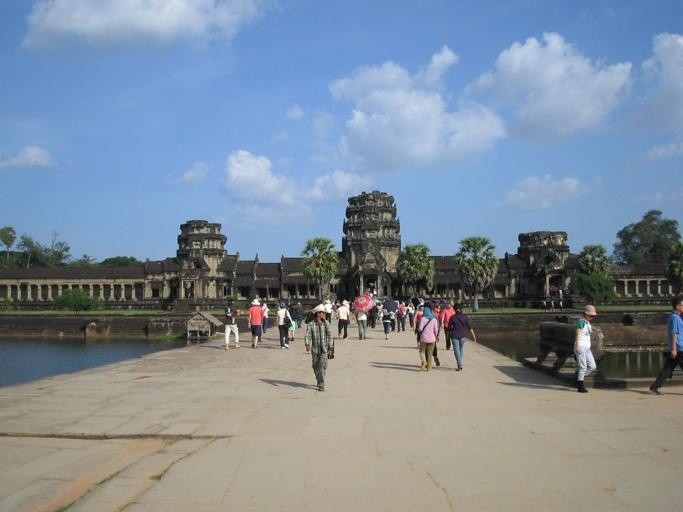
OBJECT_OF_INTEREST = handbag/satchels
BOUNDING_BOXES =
[284,316,292,328]
[328,339,334,359]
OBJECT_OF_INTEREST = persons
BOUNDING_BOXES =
[285,289,454,372]
[573,303,598,393]
[447,303,476,372]
[223,298,241,350]
[648,297,682,396]
[246,298,264,349]
[303,304,334,391]
[261,303,270,334]
[275,302,292,349]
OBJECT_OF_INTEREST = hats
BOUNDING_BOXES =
[251,299,260,305]
[281,303,285,306]
[316,307,327,312]
[325,300,331,304]
[584,305,597,316]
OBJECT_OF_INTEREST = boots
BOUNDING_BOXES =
[577,381,588,392]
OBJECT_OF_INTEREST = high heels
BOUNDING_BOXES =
[456,364,463,371]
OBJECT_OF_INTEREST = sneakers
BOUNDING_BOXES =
[319,386,324,391]
[421,362,427,370]
[288,337,294,341]
[650,386,664,395]
[434,356,440,366]
[281,344,289,349]
